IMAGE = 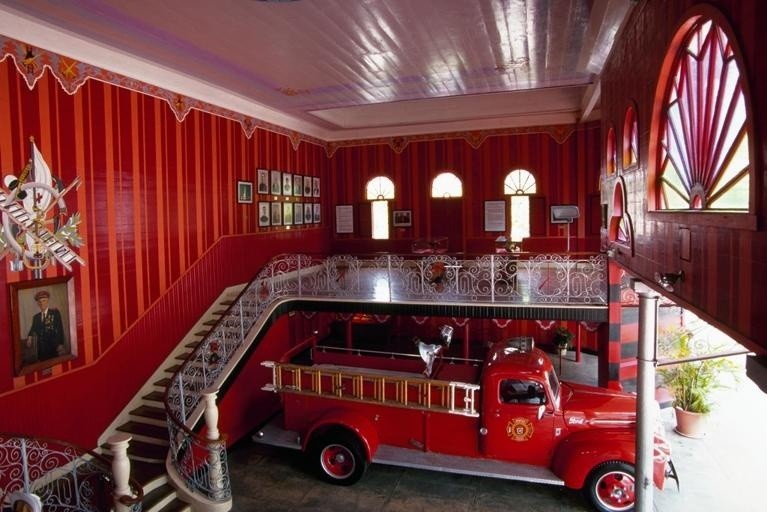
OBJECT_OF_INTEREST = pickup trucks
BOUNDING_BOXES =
[254,330,680,512]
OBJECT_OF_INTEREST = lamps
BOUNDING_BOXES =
[654,269,685,292]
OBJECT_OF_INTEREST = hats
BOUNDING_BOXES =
[34,291,50,301]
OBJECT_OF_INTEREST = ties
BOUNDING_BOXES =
[43,313,45,320]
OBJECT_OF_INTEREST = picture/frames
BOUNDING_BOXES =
[8,274,79,378]
[237,168,321,227]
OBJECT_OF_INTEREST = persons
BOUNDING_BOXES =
[26,291,64,361]
[395,212,410,223]
[502,381,527,403]
[259,173,319,223]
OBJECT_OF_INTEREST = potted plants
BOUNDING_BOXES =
[657,325,740,438]
